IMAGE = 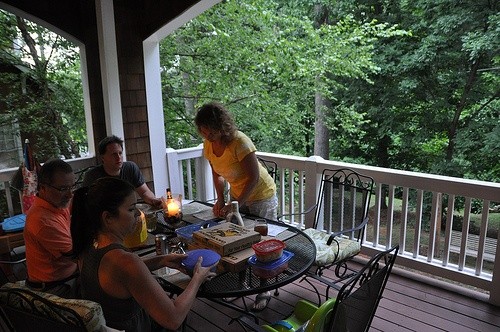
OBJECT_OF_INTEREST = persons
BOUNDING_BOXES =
[72,177,219,332]
[193,103,278,312]
[92,135,165,207]
[23,160,90,299]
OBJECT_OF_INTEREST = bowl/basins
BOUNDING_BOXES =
[180,249,220,273]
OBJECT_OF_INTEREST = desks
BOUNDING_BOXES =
[123,199,316,324]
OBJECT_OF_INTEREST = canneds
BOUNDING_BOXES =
[155,233,168,256]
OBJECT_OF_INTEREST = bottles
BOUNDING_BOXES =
[167,188,172,199]
[228,201,245,228]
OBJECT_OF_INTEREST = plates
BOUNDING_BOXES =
[181,204,204,215]
[135,203,150,211]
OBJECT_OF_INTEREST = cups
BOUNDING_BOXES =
[145,214,158,232]
[254,222,268,236]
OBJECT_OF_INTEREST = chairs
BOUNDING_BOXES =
[0,283,106,332]
[279,168,374,278]
[264,246,400,332]
[0,258,81,298]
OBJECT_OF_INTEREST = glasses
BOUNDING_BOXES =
[40,182,75,193]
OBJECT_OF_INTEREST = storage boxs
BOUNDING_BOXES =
[189,222,261,274]
[174,220,221,243]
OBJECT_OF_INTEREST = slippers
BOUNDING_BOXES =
[252,295,272,313]
